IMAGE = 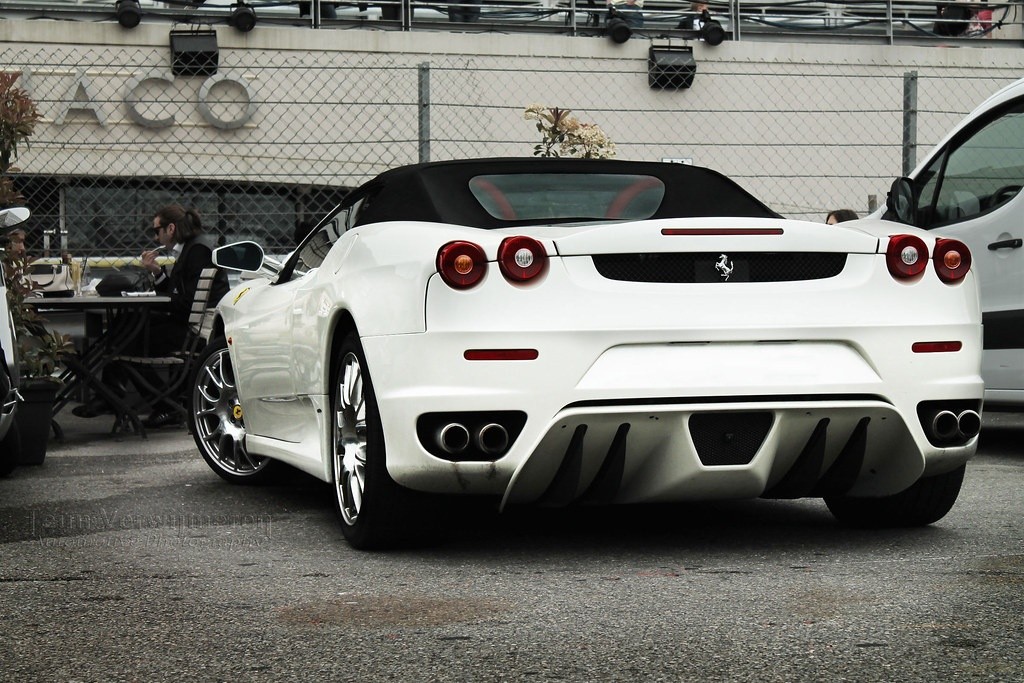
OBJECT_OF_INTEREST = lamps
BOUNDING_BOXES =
[702,19,725,46]
[229,0,257,32]
[116,0,141,29]
[608,18,632,43]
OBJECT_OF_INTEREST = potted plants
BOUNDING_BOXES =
[0,71,77,464]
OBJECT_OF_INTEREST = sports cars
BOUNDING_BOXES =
[183,155,989,553]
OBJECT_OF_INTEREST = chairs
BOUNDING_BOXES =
[105,267,218,441]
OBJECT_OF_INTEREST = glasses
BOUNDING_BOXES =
[153,226,164,235]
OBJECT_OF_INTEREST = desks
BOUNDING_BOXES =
[22,296,171,439]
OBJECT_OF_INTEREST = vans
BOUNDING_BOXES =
[863,75,1024,414]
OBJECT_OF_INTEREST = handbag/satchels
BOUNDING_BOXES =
[95,264,157,296]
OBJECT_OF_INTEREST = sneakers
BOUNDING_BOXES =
[140,402,187,428]
[72,395,114,418]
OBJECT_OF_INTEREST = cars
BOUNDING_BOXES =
[0,258,25,480]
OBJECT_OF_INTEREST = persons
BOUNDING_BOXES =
[826,209,860,227]
[70,204,231,428]
[607,0,994,40]
[1,230,27,260]
[297,0,484,24]
